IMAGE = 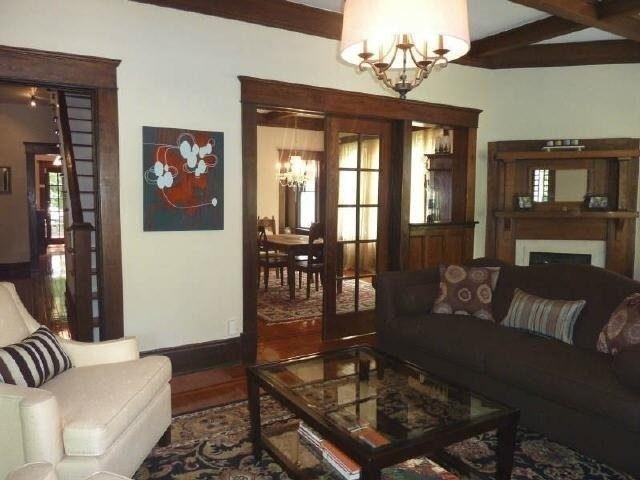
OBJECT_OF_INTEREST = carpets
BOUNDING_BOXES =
[129,360,639,480]
[255,261,376,327]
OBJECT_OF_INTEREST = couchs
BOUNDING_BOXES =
[366,258,638,480]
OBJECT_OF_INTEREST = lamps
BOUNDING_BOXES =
[337,0,473,104]
[29,96,36,108]
[273,104,323,200]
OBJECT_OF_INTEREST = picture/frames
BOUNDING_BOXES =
[514,193,532,210]
[0,165,12,195]
[584,191,612,211]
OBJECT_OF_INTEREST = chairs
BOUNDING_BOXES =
[0,280,172,479]
[254,212,345,303]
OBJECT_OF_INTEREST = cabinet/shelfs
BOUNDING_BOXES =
[491,149,640,241]
[423,152,454,223]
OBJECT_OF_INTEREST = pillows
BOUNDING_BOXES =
[1,318,75,386]
[429,260,503,325]
[498,283,586,347]
[594,288,639,352]
